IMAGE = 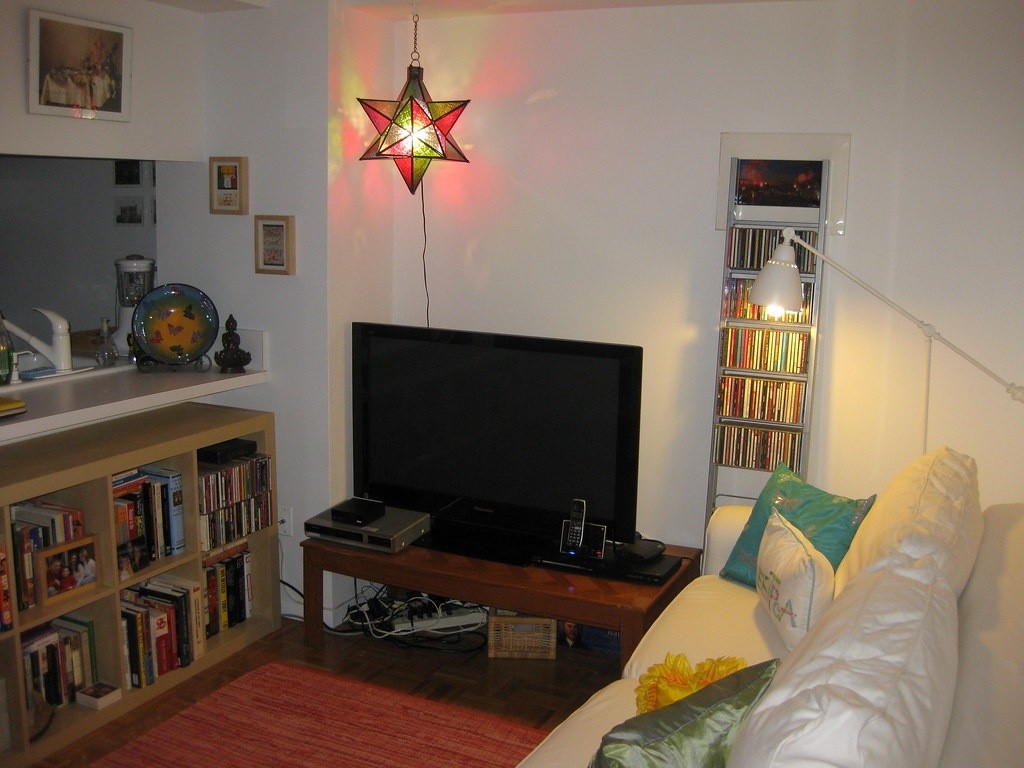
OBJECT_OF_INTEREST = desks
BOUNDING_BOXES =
[300,537,703,674]
[0,328,264,448]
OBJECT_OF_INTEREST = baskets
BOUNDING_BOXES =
[487,605,557,660]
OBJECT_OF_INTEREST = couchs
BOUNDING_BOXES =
[517,446,1024,768]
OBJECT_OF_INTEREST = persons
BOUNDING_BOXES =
[119,555,130,580]
[69,554,84,587]
[47,560,60,597]
[557,622,591,652]
[126,541,135,563]
[132,545,146,573]
[79,550,96,586]
[60,566,75,591]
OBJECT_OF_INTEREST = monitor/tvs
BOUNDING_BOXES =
[351,320,644,563]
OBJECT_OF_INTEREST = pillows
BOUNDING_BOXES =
[754,507,834,652]
[719,460,876,588]
[586,652,779,768]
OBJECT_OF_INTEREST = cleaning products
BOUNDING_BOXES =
[94,318,119,367]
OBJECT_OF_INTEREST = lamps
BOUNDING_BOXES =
[749,226,1024,454]
[357,0,470,195]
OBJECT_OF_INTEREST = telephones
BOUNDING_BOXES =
[557,498,608,560]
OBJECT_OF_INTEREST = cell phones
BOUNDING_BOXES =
[568,500,586,549]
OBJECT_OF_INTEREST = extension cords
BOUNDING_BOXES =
[391,607,486,632]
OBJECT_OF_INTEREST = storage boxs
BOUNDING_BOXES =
[557,621,620,655]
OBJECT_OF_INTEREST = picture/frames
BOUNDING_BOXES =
[254,215,295,275]
[208,157,247,215]
[27,9,134,123]
[715,133,851,235]
[113,196,144,227]
[113,160,142,187]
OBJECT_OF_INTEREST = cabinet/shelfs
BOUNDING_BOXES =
[0,402,281,768]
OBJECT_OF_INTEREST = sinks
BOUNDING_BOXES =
[6,350,97,380]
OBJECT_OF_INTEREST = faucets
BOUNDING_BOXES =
[2,306,95,380]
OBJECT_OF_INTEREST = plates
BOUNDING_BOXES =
[131,282,220,365]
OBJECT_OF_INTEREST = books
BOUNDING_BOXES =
[121,574,205,688]
[203,550,252,638]
[715,226,815,474]
[21,615,121,719]
[0,466,185,630]
[199,452,272,553]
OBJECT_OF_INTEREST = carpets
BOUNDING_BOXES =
[83,660,553,768]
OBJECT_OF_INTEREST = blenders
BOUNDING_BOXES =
[107,254,158,356]
